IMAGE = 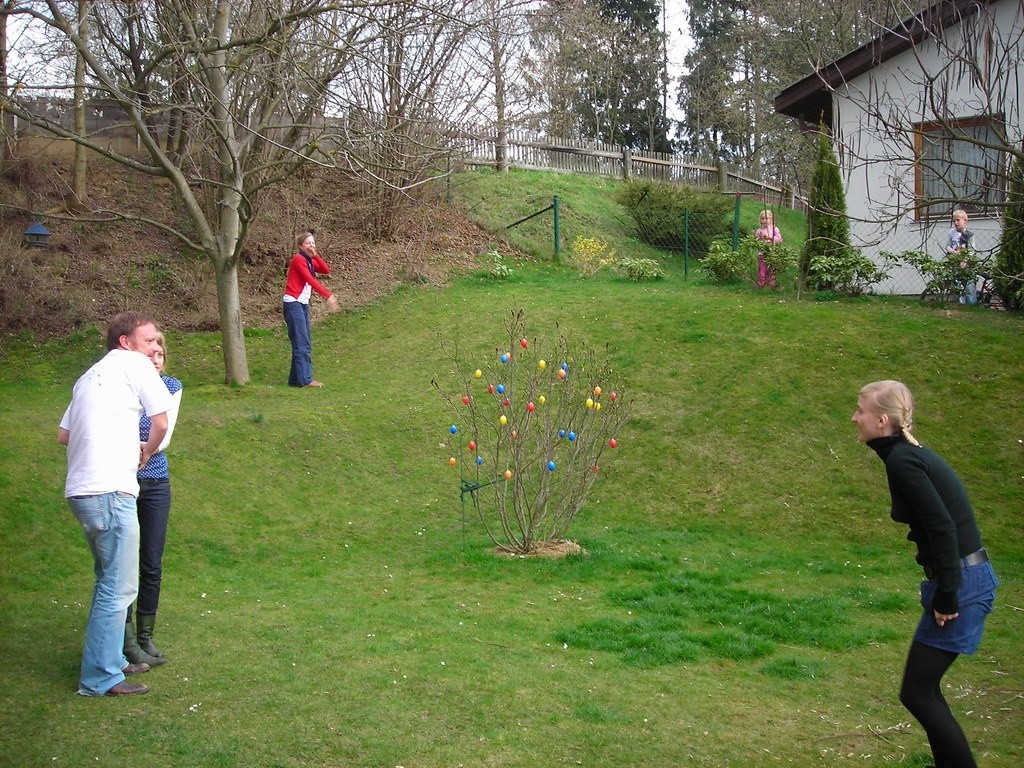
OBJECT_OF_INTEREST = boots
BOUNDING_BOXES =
[123,623,163,667]
[136,611,162,657]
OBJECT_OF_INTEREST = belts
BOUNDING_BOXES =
[924,549,988,581]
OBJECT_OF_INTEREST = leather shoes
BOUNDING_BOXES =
[122,663,150,676]
[105,679,149,696]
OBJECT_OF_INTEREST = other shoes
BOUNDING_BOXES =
[304,380,324,387]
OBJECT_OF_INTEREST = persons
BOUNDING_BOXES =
[947,210,977,305]
[851,380,999,768]
[755,209,783,289]
[59,309,183,696]
[282,232,338,388]
